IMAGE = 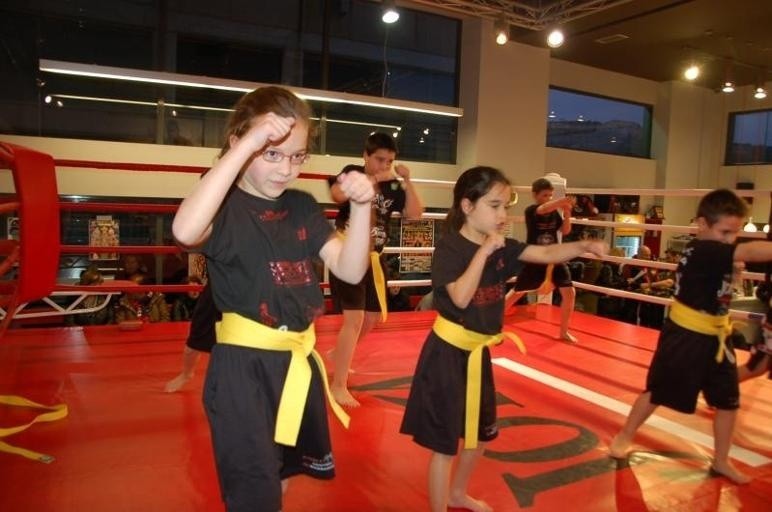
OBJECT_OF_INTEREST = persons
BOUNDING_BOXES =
[595,242,687,331]
[323,135,425,410]
[501,177,583,346]
[160,274,226,400]
[385,269,434,310]
[601,187,772,487]
[60,240,203,323]
[167,82,377,510]
[393,163,618,511]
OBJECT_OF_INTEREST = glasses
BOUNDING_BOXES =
[259,148,309,165]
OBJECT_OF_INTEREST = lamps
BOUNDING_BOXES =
[721,62,736,93]
[752,70,768,100]
[382,0,399,24]
[37,57,463,120]
[494,13,508,45]
[546,18,566,48]
